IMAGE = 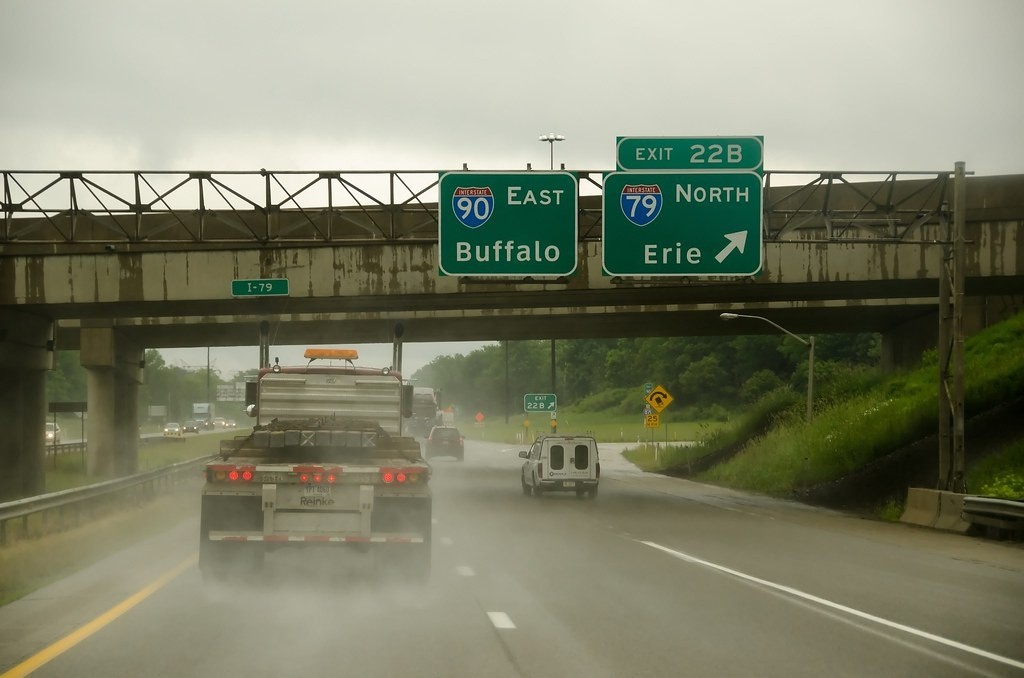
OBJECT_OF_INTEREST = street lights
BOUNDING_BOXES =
[720,312,815,426]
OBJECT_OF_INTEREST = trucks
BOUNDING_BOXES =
[398,387,439,438]
[199,367,434,592]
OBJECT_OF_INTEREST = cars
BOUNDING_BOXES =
[164,417,237,438]
[45,422,61,445]
[425,426,466,462]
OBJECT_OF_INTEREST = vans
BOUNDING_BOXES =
[519,436,600,498]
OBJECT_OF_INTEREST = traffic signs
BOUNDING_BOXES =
[601,173,765,278]
[437,172,582,275]
